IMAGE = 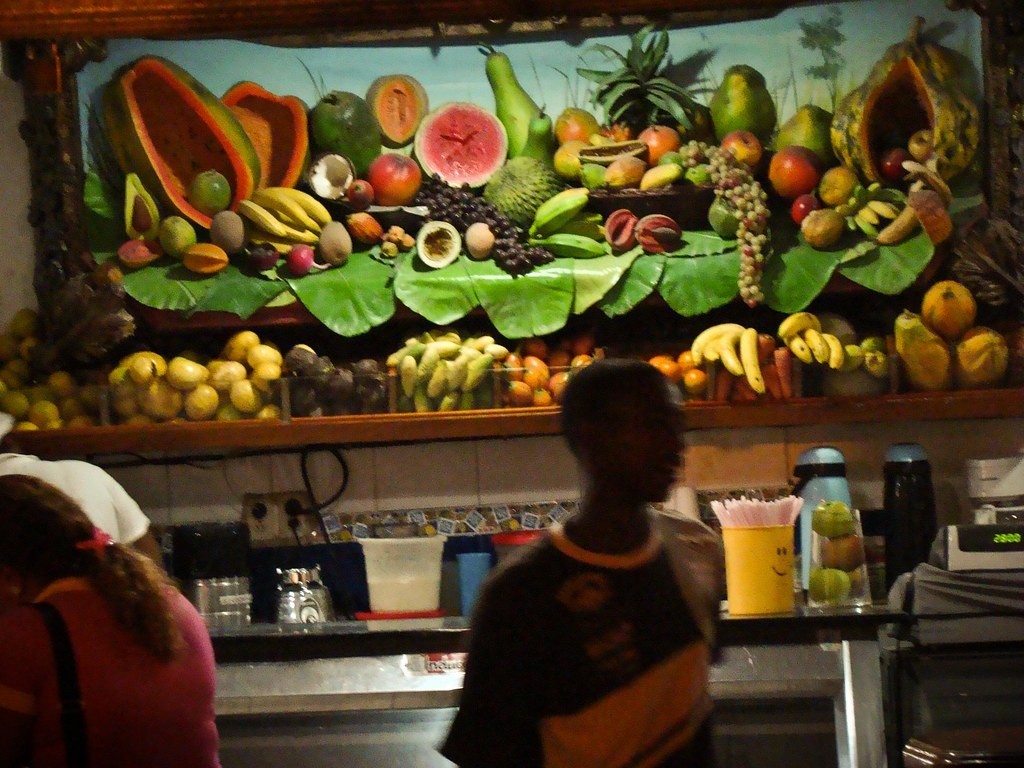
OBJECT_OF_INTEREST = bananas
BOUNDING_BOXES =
[835,161,951,245]
[689,309,844,393]
[525,187,609,258]
[235,186,332,254]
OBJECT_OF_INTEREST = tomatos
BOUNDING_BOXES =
[498,332,704,407]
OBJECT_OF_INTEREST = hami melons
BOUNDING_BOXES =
[366,72,428,145]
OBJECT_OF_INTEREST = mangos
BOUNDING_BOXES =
[385,328,509,413]
[110,327,286,422]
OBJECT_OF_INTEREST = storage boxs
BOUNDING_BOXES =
[0,357,901,436]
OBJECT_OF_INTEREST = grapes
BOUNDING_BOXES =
[412,174,556,273]
[673,137,772,308]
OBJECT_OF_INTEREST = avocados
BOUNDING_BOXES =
[123,172,161,241]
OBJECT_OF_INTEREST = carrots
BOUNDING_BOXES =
[714,334,792,402]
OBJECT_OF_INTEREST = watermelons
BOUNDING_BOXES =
[411,102,510,191]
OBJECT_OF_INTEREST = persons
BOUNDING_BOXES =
[0,412,165,570]
[0,474,224,768]
[435,359,729,768]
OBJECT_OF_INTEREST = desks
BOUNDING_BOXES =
[204,605,916,767]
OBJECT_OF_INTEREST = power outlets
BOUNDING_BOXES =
[245,495,278,540]
[280,492,308,538]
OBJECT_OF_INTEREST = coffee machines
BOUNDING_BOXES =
[965,455,1024,524]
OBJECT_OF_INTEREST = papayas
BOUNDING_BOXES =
[895,279,1009,392]
[553,18,982,192]
[102,51,379,243]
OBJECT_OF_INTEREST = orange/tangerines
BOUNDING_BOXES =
[159,168,231,259]
[0,308,104,432]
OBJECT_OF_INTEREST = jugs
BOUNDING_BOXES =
[790,446,852,602]
[883,442,936,596]
[273,564,336,623]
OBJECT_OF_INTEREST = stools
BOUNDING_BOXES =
[902,726,1024,768]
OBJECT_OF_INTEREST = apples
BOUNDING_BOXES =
[808,501,864,605]
[724,126,934,211]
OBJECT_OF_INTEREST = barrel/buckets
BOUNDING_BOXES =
[174,522,252,625]
[358,537,445,612]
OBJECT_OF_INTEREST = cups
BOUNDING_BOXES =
[456,553,490,615]
[721,526,795,616]
